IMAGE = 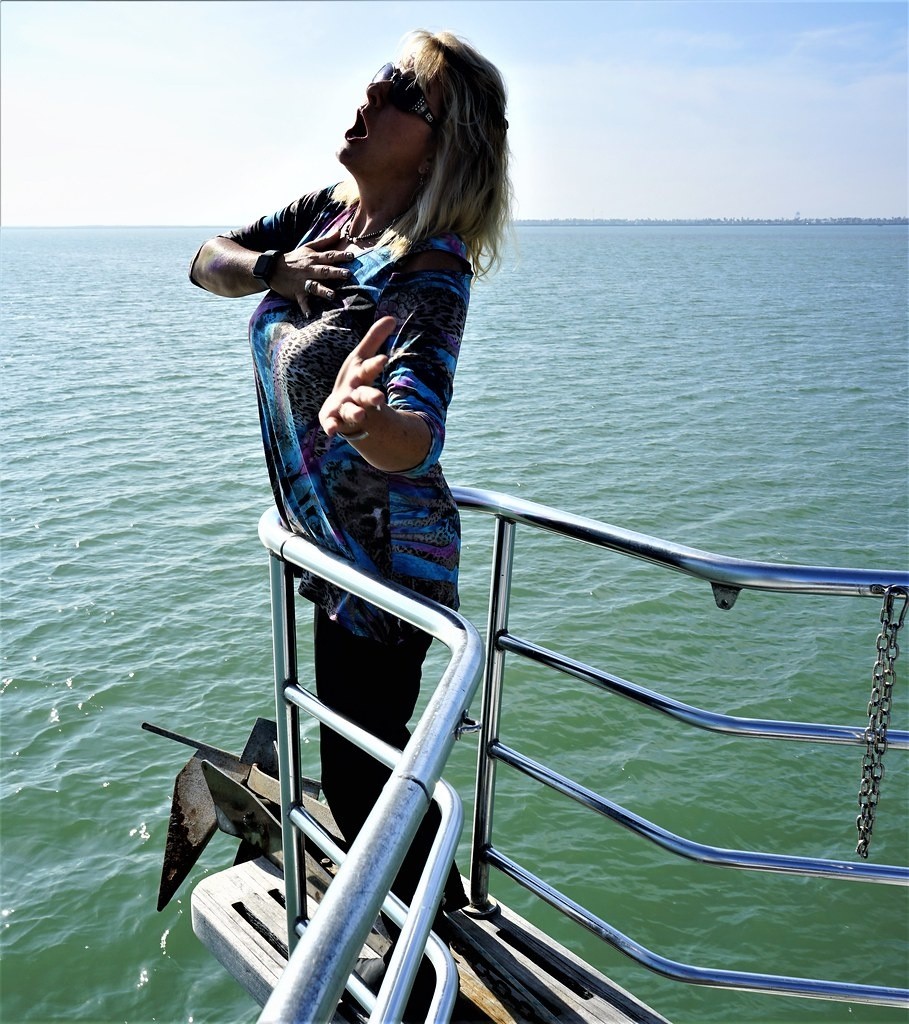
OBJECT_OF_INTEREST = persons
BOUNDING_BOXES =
[186,24,507,1010]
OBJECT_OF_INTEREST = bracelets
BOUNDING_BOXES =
[336,404,382,443]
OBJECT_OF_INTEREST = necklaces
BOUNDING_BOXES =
[337,205,410,261]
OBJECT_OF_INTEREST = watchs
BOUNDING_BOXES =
[251,248,282,290]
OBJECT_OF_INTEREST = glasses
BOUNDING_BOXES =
[371,62,440,132]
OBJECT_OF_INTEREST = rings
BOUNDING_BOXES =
[304,279,313,293]
[337,402,345,414]
